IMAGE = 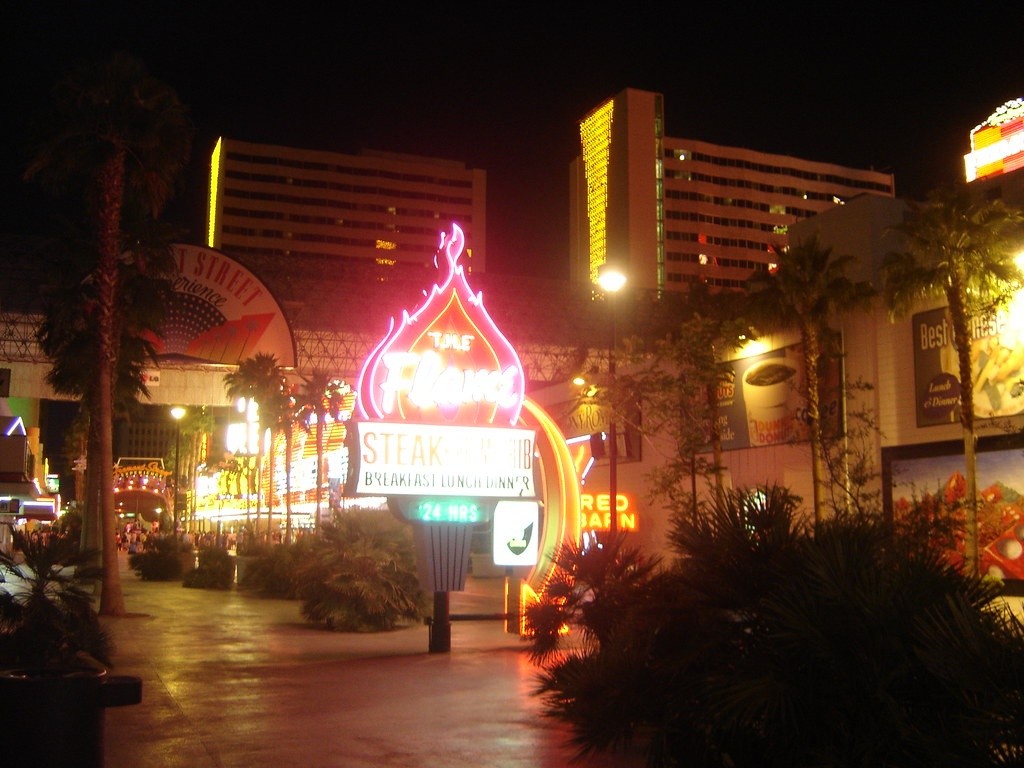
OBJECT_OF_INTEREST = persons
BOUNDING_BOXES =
[117,518,317,555]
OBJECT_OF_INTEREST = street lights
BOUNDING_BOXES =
[170,405,185,534]
[600,266,627,538]
